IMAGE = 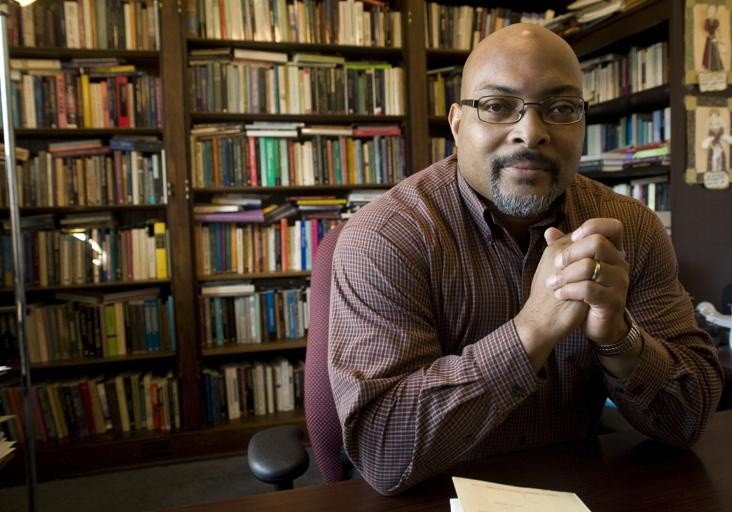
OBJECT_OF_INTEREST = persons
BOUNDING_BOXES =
[326,20,727,498]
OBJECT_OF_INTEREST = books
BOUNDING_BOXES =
[446,476,591,512]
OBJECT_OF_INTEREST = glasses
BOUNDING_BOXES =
[460,94,591,130]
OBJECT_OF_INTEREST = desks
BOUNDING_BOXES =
[160,409,731,512]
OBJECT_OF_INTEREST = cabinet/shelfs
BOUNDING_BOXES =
[418,0,732,325]
[1,0,424,489]
